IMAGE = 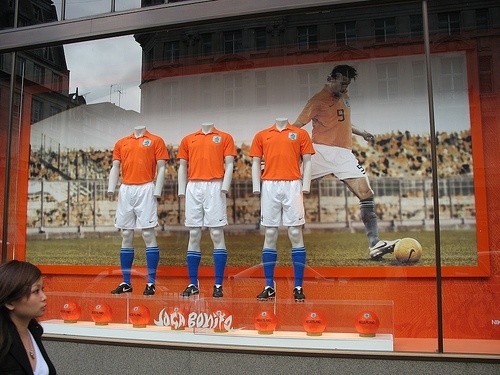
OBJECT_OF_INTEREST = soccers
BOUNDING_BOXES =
[394,238,423,264]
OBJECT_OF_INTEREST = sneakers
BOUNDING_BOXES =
[181,284,199,296]
[295,288,305,300]
[111,282,132,294]
[257,287,275,300]
[213,285,223,297]
[143,284,156,295]
[369,239,400,260]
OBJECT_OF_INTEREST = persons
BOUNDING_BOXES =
[27,64,474,258]
[176,123,237,297]
[249,118,316,302]
[106,126,170,295]
[0,260,58,375]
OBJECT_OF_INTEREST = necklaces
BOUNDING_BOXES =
[28,342,36,360]
